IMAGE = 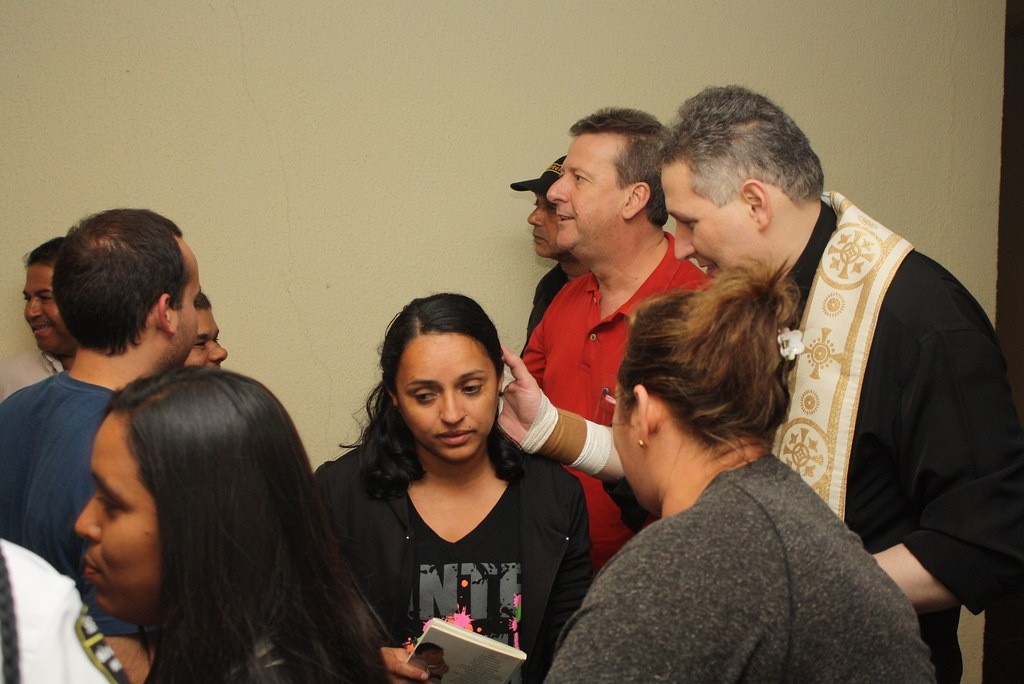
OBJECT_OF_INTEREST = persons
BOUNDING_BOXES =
[72,365,404,684]
[494,88,1024,684]
[309,289,596,684]
[517,107,714,574]
[0,208,227,684]
[543,256,939,683]
[512,154,590,361]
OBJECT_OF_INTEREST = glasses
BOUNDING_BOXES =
[597,388,616,413]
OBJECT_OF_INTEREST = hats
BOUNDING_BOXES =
[510,155,569,195]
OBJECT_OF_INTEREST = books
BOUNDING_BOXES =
[406,617,527,684]
